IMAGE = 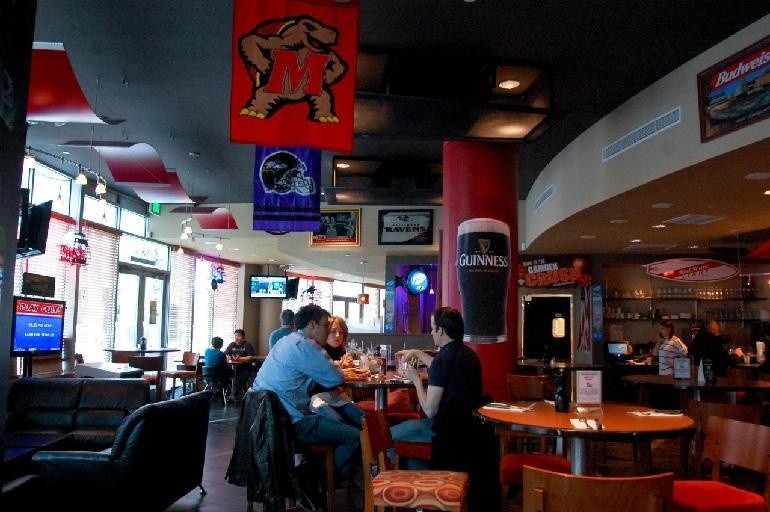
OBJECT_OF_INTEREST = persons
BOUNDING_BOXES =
[650,320,688,411]
[686,321,735,379]
[204,336,233,406]
[250,305,366,511]
[384,307,481,471]
[223,329,256,404]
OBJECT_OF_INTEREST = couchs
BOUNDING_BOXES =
[32,390,212,511]
[2,376,84,508]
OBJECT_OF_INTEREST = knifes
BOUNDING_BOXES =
[595,417,603,431]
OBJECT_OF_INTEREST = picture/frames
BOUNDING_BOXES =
[696,34,770,143]
[377,208,434,245]
[308,208,361,247]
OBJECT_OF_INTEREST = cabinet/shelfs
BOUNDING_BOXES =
[601,296,767,327]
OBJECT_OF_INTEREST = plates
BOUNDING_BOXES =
[661,312,692,320]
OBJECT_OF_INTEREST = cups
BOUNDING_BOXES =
[606,307,640,320]
[456,219,512,344]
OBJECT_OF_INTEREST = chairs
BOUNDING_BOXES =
[522,463,672,510]
[494,422,575,506]
[244,390,337,511]
[672,413,769,511]
[389,417,435,469]
[359,408,468,512]
[2,353,770,409]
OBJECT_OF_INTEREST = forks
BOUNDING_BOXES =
[579,412,593,430]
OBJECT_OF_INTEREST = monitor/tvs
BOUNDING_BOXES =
[248,275,288,297]
[15,199,53,262]
[286,276,300,300]
[10,296,65,358]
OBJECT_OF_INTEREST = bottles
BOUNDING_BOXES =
[652,286,760,300]
[360,350,380,373]
[554,384,569,412]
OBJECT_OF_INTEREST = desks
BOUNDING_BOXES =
[104,349,180,355]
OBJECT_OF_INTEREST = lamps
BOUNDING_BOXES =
[357,261,369,304]
[24,76,223,250]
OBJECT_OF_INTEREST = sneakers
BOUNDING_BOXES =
[297,478,319,511]
